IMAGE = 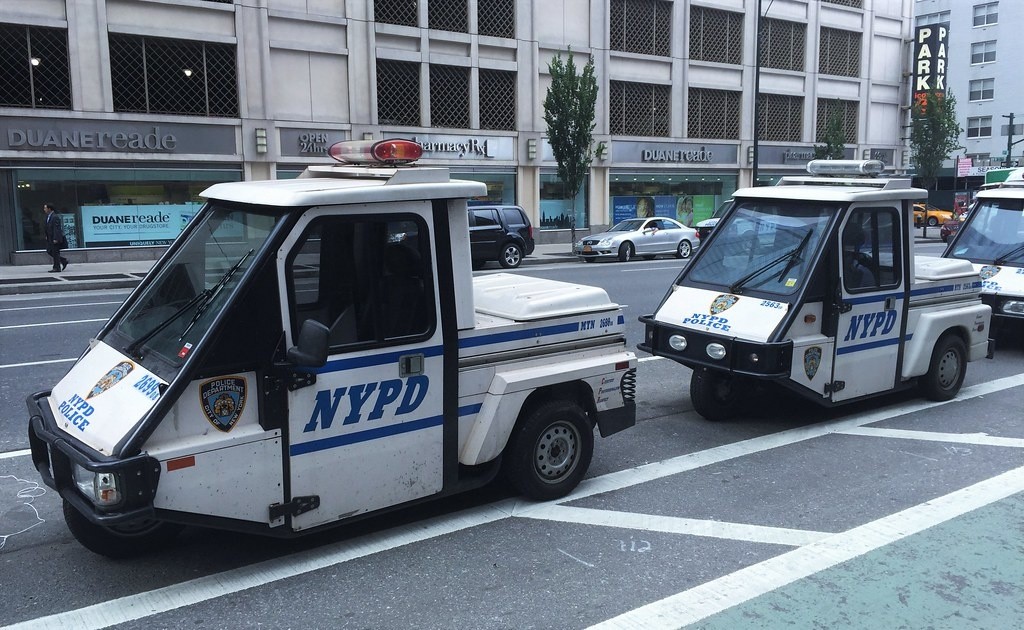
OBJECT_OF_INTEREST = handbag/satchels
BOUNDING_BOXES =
[59,235,69,248]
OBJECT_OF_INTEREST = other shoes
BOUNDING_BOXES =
[62,261,68,271]
[48,268,61,272]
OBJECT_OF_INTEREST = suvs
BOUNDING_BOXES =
[466,205,536,270]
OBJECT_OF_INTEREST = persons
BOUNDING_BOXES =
[676,196,693,228]
[651,222,659,231]
[636,197,655,217]
[846,255,875,288]
[43,203,68,272]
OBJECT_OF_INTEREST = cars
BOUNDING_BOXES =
[572,215,701,263]
[638,158,993,421]
[913,203,954,227]
[22,139,640,561]
[939,201,975,243]
[943,168,1024,331]
[697,198,736,240]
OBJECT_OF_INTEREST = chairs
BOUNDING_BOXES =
[318,239,428,347]
[842,223,881,287]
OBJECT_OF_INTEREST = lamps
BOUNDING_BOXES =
[863,149,871,160]
[748,145,754,162]
[902,151,910,165]
[600,141,608,160]
[528,139,540,159]
[255,128,268,155]
